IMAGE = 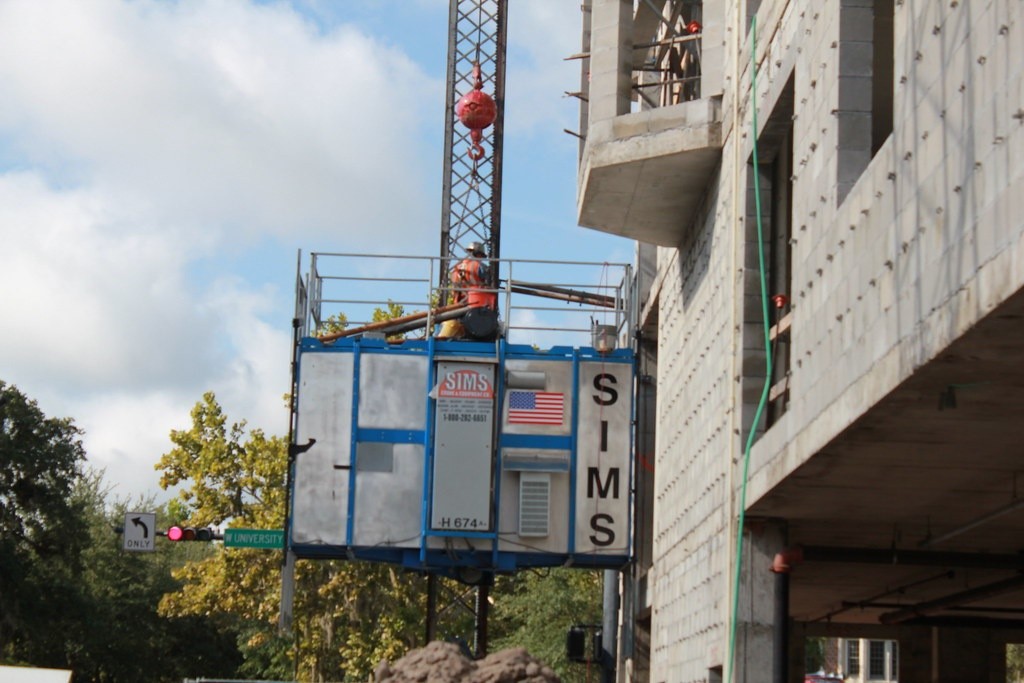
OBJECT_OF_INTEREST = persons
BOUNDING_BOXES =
[437,240,493,339]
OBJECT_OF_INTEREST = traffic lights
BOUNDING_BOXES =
[168,527,213,542]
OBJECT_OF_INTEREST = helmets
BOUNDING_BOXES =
[466,242,487,259]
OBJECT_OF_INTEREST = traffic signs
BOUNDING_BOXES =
[123,512,155,551]
[223,530,285,549]
